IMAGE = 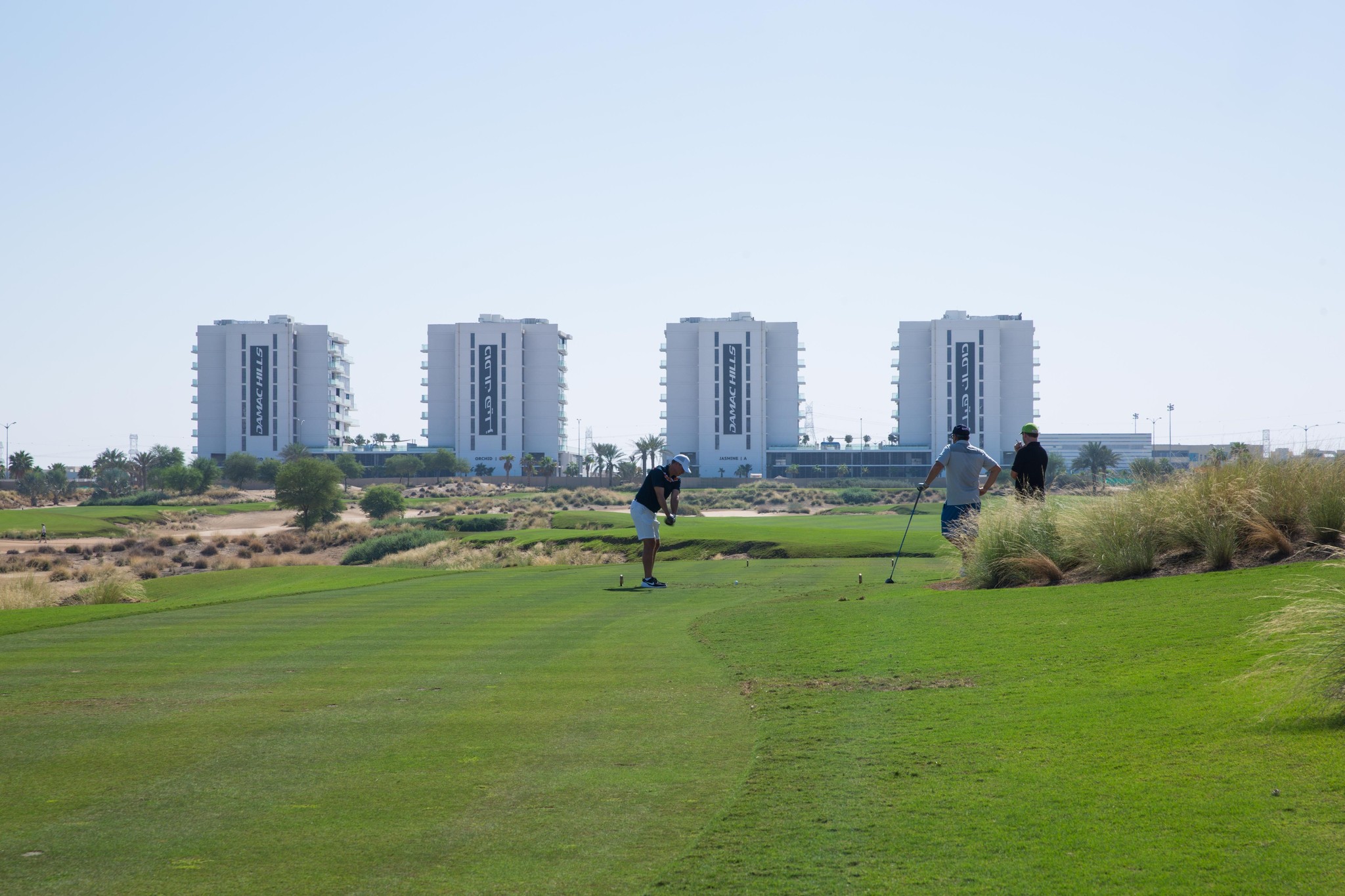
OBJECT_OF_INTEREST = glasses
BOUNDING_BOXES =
[951,435,954,439]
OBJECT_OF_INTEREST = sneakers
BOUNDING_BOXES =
[640,576,667,588]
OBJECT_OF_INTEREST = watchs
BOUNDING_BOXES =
[923,480,930,487]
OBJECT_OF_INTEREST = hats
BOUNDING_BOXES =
[673,454,691,474]
[1018,423,1038,436]
[949,423,970,436]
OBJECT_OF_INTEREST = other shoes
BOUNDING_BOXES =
[959,567,968,577]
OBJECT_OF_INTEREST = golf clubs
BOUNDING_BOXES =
[884,486,924,584]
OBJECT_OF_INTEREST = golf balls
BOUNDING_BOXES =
[734,581,738,584]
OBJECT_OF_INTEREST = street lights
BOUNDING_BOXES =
[860,418,862,476]
[0,422,16,479]
[1293,425,1319,458]
[1133,413,1139,433]
[1146,418,1162,460]
[577,419,581,477]
[1167,403,1174,463]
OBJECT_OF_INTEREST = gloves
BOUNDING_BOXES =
[917,483,929,491]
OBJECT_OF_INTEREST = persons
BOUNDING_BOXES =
[921,424,1001,578]
[39,523,47,544]
[1011,423,1048,503]
[630,454,691,588]
